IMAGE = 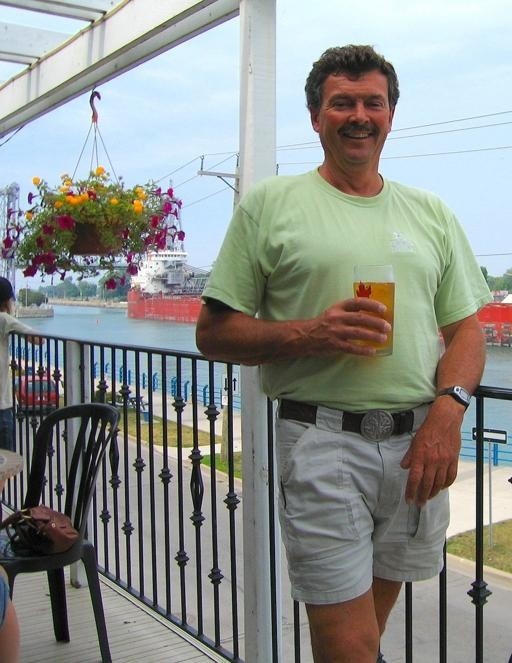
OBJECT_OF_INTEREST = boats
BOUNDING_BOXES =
[128,181,207,347]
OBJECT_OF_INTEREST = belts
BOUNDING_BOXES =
[275,398,415,443]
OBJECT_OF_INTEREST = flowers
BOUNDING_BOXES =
[3,166,189,288]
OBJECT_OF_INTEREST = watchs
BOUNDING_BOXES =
[435,384,471,414]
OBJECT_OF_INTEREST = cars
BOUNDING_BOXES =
[13,375,59,415]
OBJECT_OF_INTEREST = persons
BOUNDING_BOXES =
[192,40,497,663]
[0,572,25,663]
[0,274,47,494]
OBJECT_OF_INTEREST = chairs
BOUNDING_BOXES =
[1,401,119,662]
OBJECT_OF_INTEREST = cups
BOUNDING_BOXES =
[352,264,396,358]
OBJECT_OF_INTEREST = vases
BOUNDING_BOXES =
[65,220,121,256]
